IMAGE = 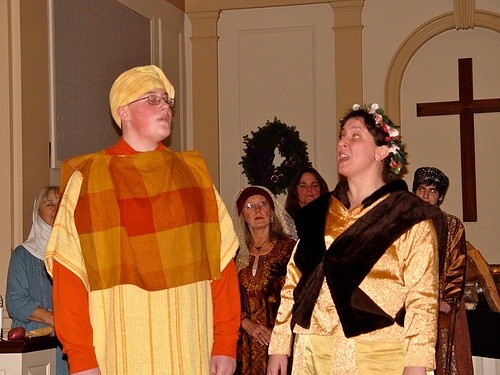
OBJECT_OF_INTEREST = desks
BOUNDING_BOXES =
[0,337,58,375]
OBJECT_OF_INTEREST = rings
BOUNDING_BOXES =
[257,332,261,337]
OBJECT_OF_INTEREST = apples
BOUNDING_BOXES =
[8,327,26,341]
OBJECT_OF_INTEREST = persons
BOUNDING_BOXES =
[412,167,474,375]
[4,185,71,375]
[285,168,329,218]
[230,185,298,375]
[45,63,242,375]
[265,104,440,375]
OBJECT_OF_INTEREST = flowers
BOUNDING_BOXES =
[339,102,408,178]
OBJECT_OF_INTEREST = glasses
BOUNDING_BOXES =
[242,201,267,209]
[124,94,175,110]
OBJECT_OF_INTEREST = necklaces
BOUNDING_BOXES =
[252,237,268,255]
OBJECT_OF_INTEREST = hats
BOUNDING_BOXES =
[236,186,275,216]
[412,167,450,196]
[108,65,177,128]
